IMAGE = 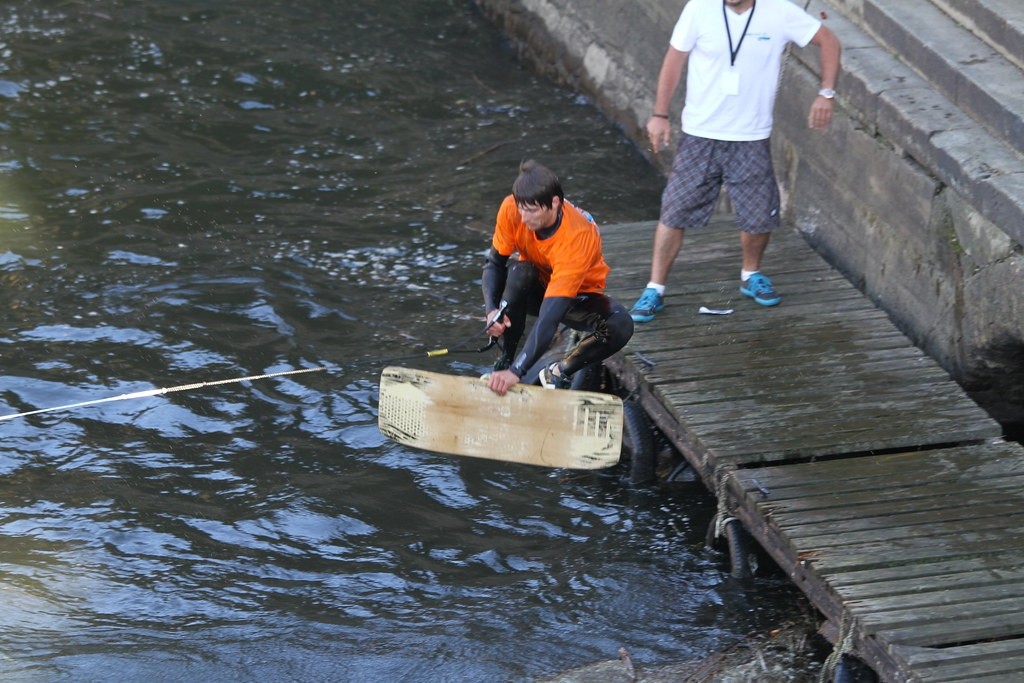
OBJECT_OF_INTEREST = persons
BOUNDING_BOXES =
[630,0,842,319]
[482,158,634,396]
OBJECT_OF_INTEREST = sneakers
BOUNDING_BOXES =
[493,356,511,372]
[539,364,565,390]
[627,287,664,320]
[740,271,781,306]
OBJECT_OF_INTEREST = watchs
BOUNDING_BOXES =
[818,88,837,99]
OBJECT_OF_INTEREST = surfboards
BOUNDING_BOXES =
[377,365,626,472]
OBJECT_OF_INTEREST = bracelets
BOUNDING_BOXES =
[652,113,669,119]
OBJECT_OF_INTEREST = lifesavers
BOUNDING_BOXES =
[704,512,752,581]
[622,398,654,481]
[521,341,601,392]
